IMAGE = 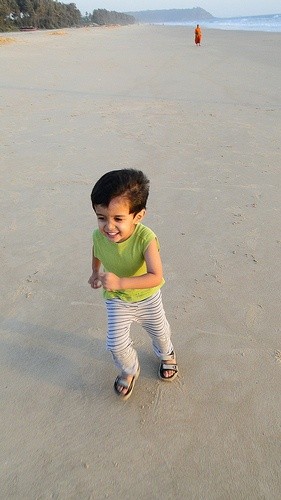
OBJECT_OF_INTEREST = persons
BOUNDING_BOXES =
[194,24,202,47]
[88,169,179,399]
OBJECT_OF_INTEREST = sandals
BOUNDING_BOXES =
[113,362,140,400]
[157,350,179,381]
